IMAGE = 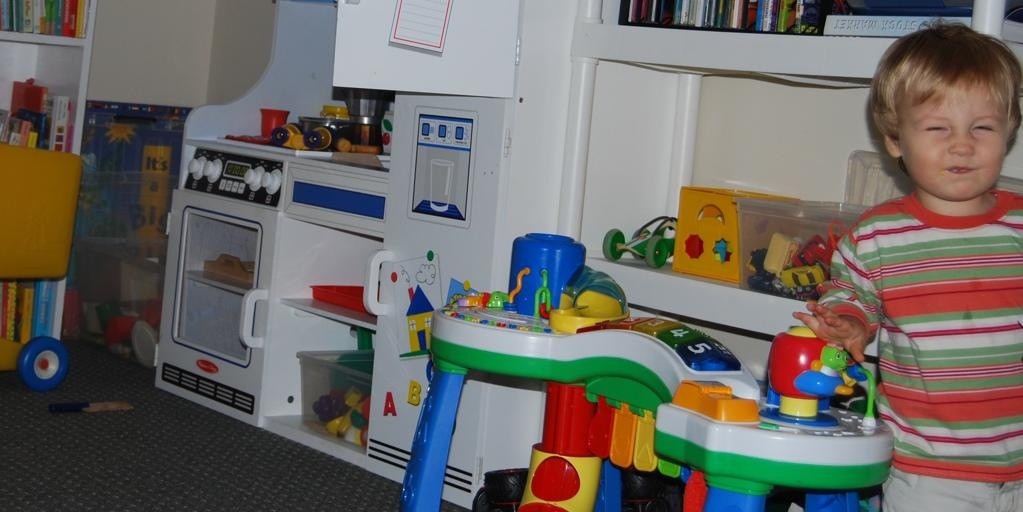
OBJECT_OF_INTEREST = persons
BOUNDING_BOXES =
[792,21,1021,511]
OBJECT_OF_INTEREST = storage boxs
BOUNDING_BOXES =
[727,196,876,302]
[296,350,374,456]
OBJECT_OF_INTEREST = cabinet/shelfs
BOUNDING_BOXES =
[554,0,1023,394]
[0,0,96,337]
[260,213,383,468]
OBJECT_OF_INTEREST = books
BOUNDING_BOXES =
[0,0,88,351]
